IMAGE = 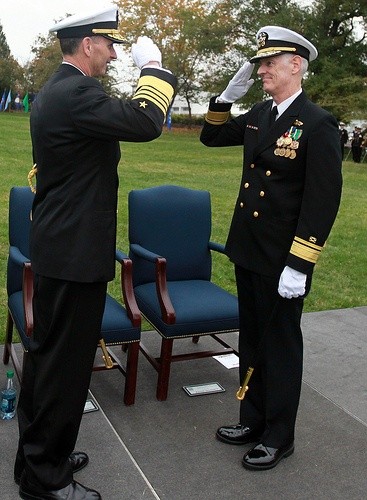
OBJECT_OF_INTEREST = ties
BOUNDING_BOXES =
[268,106,278,127]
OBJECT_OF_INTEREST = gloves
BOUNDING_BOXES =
[131,35,162,70]
[277,267,306,299]
[218,62,255,103]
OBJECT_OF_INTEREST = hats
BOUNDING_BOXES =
[249,26,318,64]
[339,122,346,126]
[49,5,130,43]
[357,126,362,129]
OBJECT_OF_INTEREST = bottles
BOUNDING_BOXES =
[0,370,17,420]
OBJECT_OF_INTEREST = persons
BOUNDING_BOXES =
[200,24,343,472]
[339,121,366,163]
[13,6,179,500]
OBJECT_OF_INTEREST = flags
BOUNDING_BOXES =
[167,110,171,130]
[0,88,29,113]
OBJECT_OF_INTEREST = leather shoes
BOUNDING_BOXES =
[216,423,260,444]
[242,441,294,470]
[68,452,89,474]
[18,481,101,500]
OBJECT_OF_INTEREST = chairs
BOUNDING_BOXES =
[2,187,142,405]
[125,186,239,400]
